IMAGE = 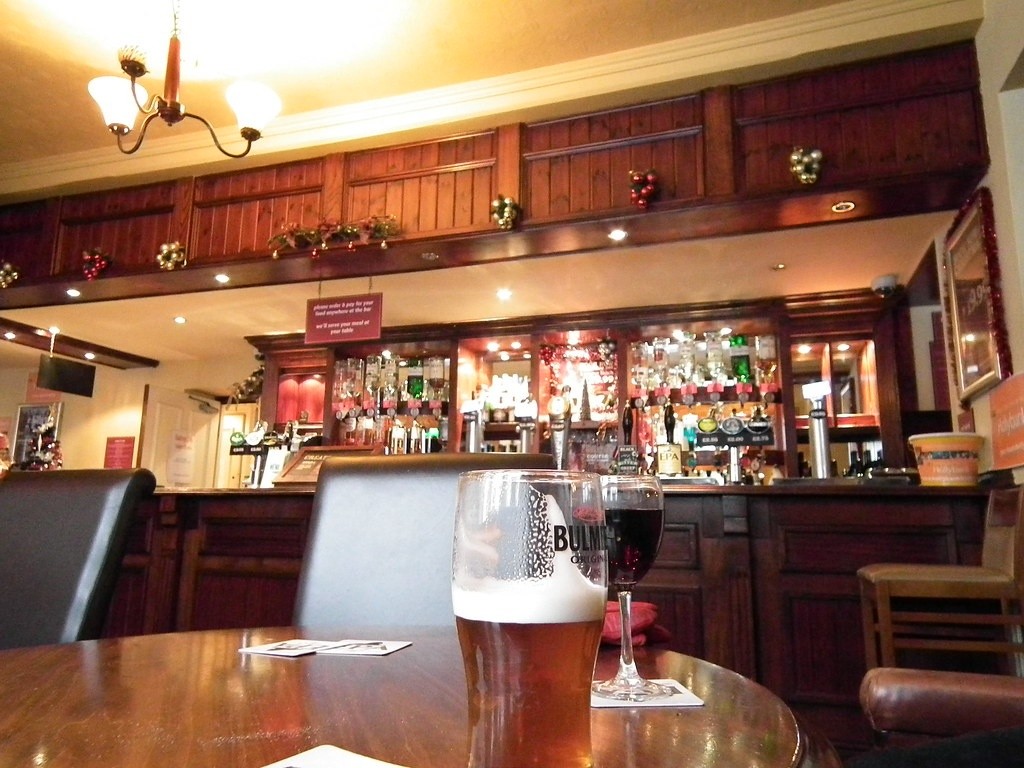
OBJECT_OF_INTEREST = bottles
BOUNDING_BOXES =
[405,356,425,399]
[652,337,669,383]
[348,356,362,398]
[704,333,724,376]
[729,336,751,382]
[756,335,777,383]
[428,355,445,401]
[441,417,448,441]
[630,341,646,394]
[676,332,696,382]
[384,353,398,397]
[366,356,381,403]
[333,360,346,400]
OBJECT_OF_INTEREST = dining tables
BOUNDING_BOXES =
[0,627,843,768]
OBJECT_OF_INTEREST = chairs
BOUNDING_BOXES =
[855,485,1023,671]
[290,450,557,642]
[0,466,157,648]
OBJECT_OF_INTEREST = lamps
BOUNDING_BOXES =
[85,0,282,160]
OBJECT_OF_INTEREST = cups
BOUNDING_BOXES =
[452,470,605,768]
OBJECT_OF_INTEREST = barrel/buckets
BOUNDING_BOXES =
[909,433,985,485]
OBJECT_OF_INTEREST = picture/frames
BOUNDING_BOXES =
[11,402,63,467]
[942,187,1014,403]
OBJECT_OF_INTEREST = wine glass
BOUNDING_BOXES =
[593,475,670,700]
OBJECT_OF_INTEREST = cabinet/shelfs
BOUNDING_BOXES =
[244,282,920,487]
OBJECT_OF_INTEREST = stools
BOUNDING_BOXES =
[857,666,1024,768]
